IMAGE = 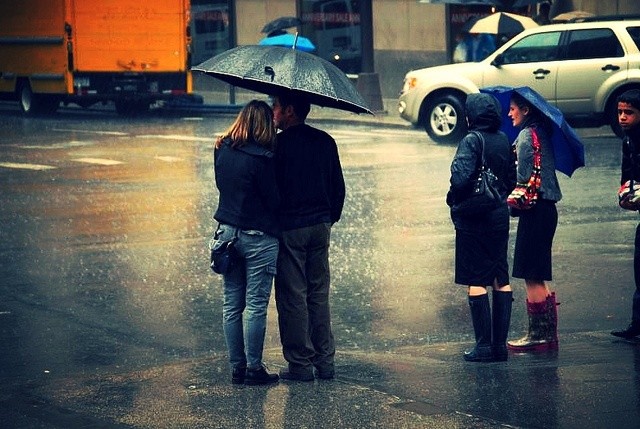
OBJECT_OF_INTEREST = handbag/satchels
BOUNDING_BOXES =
[449,130,507,223]
[207,222,240,274]
[508,127,542,212]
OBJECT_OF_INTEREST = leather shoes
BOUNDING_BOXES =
[278,364,314,382]
[244,364,279,385]
[232,366,247,384]
[608,321,639,338]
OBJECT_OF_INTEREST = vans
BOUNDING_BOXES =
[191,4,231,65]
[313,0,362,62]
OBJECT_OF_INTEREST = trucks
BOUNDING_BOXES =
[0,0,203,115]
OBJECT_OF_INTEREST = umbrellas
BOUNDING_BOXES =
[468,11,539,36]
[189,44,375,116]
[552,10,596,20]
[259,17,308,34]
[479,86,585,178]
[260,34,315,51]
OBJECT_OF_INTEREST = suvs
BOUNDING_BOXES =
[398,15,639,143]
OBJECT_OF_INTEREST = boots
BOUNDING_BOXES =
[506,300,551,352]
[463,293,494,362]
[491,289,515,360]
[545,292,559,351]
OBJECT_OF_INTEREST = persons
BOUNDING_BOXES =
[447,93,517,363]
[506,91,561,352]
[610,89,640,345]
[271,90,345,383]
[214,100,294,386]
